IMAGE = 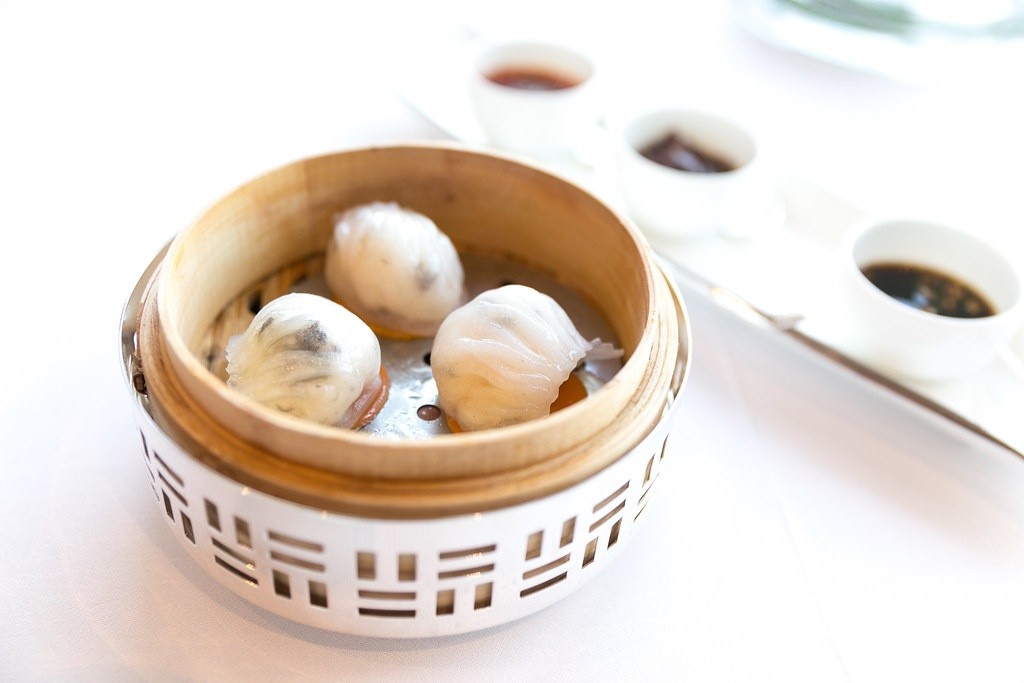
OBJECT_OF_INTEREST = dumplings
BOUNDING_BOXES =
[222,198,627,434]
